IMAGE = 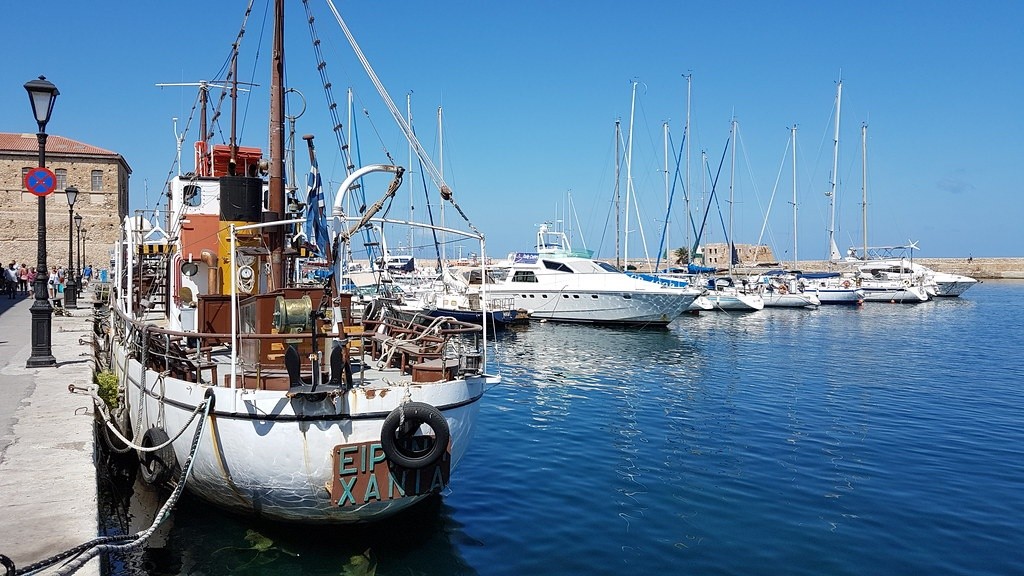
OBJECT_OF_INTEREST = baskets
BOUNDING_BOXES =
[82,282,87,286]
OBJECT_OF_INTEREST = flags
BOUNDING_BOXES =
[306,145,330,257]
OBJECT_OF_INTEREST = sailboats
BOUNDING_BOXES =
[605,68,978,313]
[101,0,501,522]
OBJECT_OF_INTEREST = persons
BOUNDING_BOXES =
[84,265,92,289]
[48,266,58,299]
[0,260,19,294]
[19,264,28,295]
[27,267,37,299]
[47,266,65,292]
[3,264,19,299]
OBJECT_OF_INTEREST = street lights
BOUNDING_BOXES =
[24,76,60,367]
[81,228,87,272]
[74,213,82,290]
[64,185,77,308]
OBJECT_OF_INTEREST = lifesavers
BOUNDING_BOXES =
[360,299,381,329]
[779,285,787,294]
[381,402,450,469]
[903,279,911,287]
[844,280,850,286]
[140,428,176,485]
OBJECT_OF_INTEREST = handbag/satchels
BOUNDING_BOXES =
[49,280,54,284]
[58,284,63,293]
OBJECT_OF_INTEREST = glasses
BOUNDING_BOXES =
[53,268,56,269]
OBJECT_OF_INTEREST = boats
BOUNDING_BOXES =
[346,89,706,333]
